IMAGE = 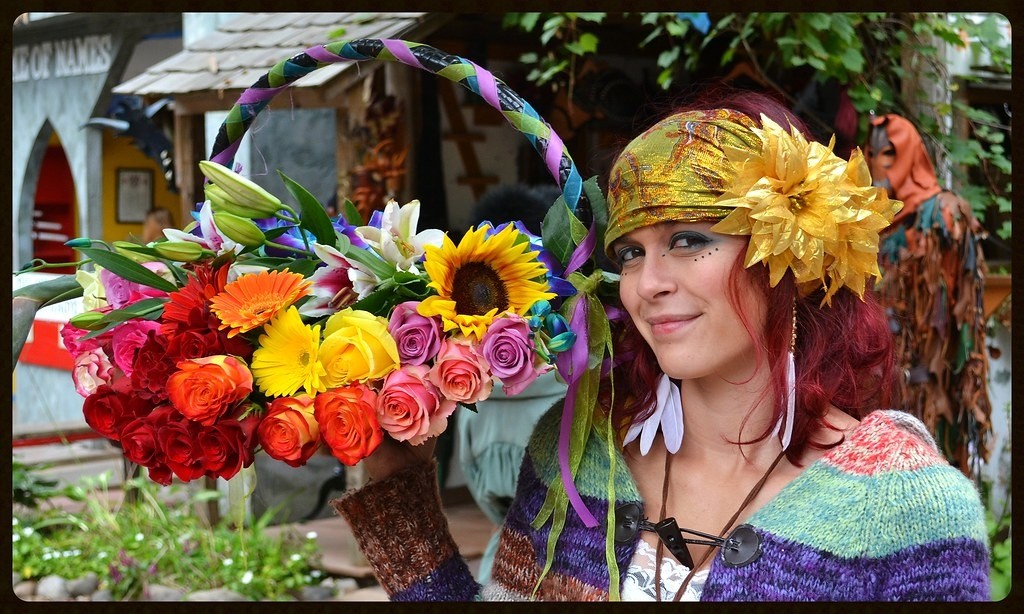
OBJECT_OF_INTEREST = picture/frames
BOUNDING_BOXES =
[116,166,154,224]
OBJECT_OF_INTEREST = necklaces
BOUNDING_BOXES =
[655,445,785,603]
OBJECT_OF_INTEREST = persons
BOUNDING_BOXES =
[852,113,998,477]
[142,208,175,245]
[329,86,992,603]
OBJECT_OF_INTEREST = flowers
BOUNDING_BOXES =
[713,115,902,308]
[62,159,569,491]
